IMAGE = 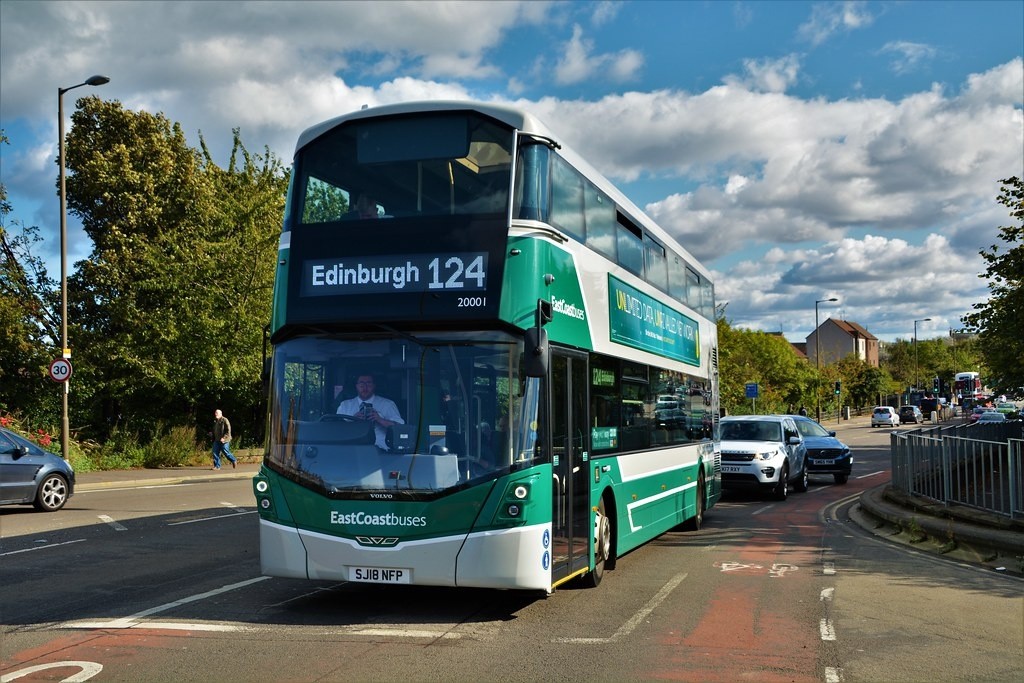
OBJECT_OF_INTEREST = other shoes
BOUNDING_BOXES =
[211,467,220,470]
[233,461,237,469]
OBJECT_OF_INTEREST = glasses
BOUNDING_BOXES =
[356,381,374,387]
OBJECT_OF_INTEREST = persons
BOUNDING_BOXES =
[787,403,794,414]
[211,410,238,469]
[799,403,807,416]
[336,374,405,452]
[351,192,384,218]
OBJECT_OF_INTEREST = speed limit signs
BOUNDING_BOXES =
[49,357,73,384]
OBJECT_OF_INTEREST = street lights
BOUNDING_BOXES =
[815,297,839,423]
[54,73,112,459]
[914,318,932,388]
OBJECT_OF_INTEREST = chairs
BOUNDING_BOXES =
[641,426,668,444]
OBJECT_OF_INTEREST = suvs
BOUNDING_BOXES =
[720,414,811,503]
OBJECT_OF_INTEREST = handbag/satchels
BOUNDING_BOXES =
[802,408,807,416]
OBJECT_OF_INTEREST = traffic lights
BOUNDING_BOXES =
[933,379,940,394]
[835,381,841,395]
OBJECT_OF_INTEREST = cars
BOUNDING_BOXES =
[0,427,76,512]
[789,415,855,484]
[656,379,711,438]
[870,406,901,427]
[969,394,1019,422]
[898,405,924,424]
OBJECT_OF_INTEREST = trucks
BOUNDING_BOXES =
[919,398,946,418]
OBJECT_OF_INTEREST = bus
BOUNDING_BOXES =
[954,371,982,404]
[249,100,726,600]
